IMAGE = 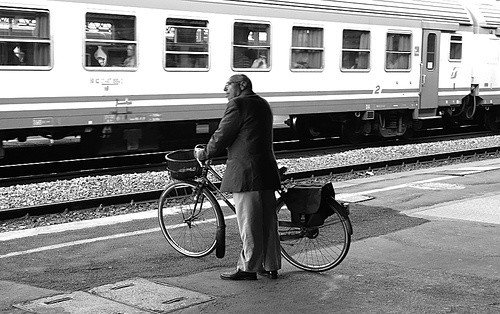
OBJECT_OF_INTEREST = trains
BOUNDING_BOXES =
[0,0,500,175]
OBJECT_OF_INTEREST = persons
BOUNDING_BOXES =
[250,52,267,68]
[195,73,282,281]
[120,44,136,67]
[351,57,359,70]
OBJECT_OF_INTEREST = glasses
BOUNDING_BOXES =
[226,82,240,86]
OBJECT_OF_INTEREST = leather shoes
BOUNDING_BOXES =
[258,270,278,279]
[220,269,257,280]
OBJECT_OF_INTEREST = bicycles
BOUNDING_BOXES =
[157,142,353,272]
